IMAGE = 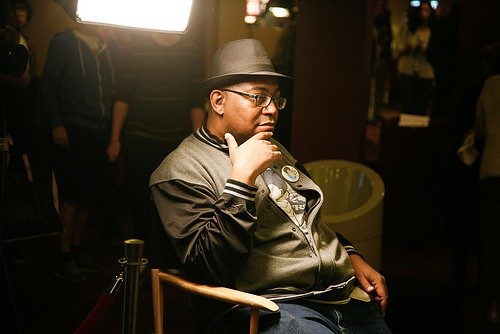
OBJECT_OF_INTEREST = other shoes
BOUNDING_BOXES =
[77,255,101,273]
[55,260,88,282]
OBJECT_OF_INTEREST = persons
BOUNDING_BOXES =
[37,23,187,282]
[0,0,36,180]
[149,38,389,334]
[374,0,500,334]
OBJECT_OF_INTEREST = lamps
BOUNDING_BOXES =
[51,0,194,34]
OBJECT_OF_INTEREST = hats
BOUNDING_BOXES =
[201,38,295,90]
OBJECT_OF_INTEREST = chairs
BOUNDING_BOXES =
[130,140,280,333]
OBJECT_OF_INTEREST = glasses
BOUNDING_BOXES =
[219,87,287,109]
